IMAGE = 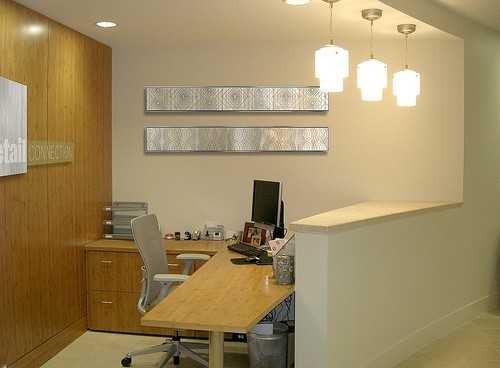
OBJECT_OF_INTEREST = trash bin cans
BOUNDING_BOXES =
[246,321,290,368]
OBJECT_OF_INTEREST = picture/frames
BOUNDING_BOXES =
[243,222,266,243]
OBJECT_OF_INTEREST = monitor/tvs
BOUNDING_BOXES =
[251,180,285,240]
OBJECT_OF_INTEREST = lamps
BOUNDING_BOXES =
[357,9,387,101]
[393,23,421,106]
[315,0,349,92]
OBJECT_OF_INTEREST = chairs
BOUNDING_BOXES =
[121,213,209,368]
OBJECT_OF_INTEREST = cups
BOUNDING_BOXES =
[276,254,295,284]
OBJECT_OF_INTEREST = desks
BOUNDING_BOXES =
[141,239,295,368]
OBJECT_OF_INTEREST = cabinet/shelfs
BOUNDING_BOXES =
[0,0,112,368]
[84,237,234,340]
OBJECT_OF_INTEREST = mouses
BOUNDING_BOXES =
[244,256,260,263]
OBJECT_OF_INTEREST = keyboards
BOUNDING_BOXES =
[228,242,268,258]
